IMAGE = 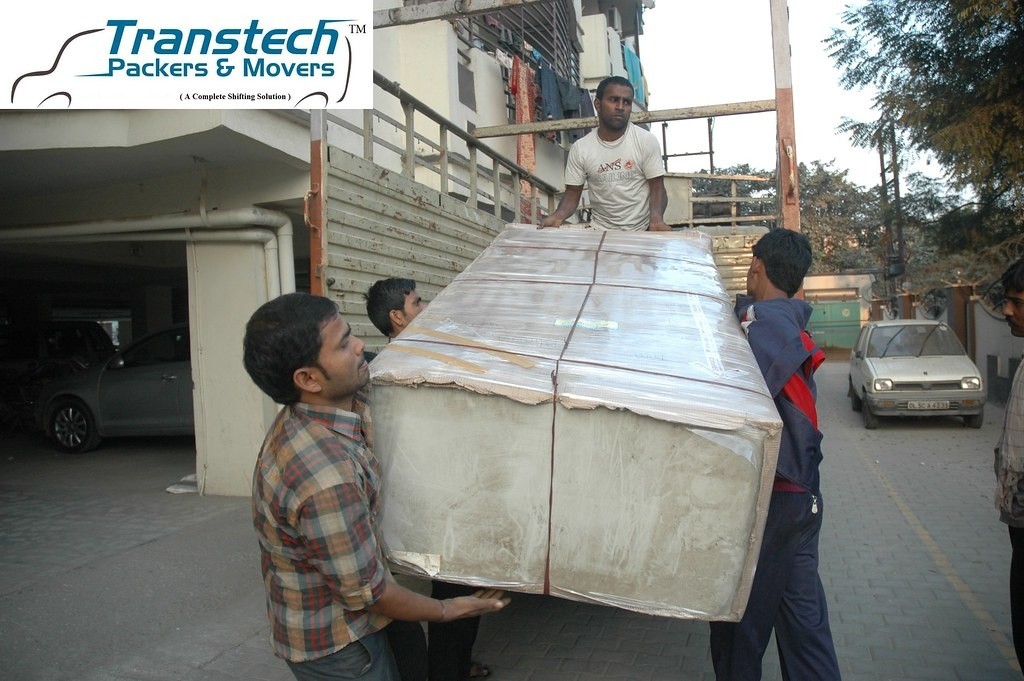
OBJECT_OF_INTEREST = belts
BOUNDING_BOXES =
[773,481,811,494]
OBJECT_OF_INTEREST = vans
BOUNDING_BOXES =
[0,320,117,367]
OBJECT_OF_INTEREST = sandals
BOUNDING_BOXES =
[470,662,493,681]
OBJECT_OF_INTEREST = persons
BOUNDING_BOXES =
[708,228,841,681]
[366,278,494,681]
[536,76,673,231]
[243,292,512,681]
[993,256,1024,676]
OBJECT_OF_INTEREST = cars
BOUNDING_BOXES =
[846,317,988,430]
[20,320,195,454]
